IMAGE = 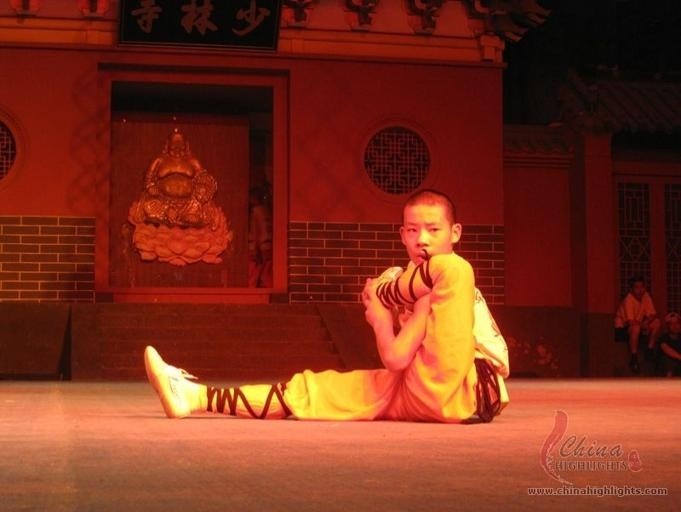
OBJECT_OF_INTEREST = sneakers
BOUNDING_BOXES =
[142,343,199,421]
[367,264,404,290]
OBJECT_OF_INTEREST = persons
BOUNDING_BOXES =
[654,311,681,377]
[614,275,662,375]
[142,189,511,424]
[144,127,218,228]
